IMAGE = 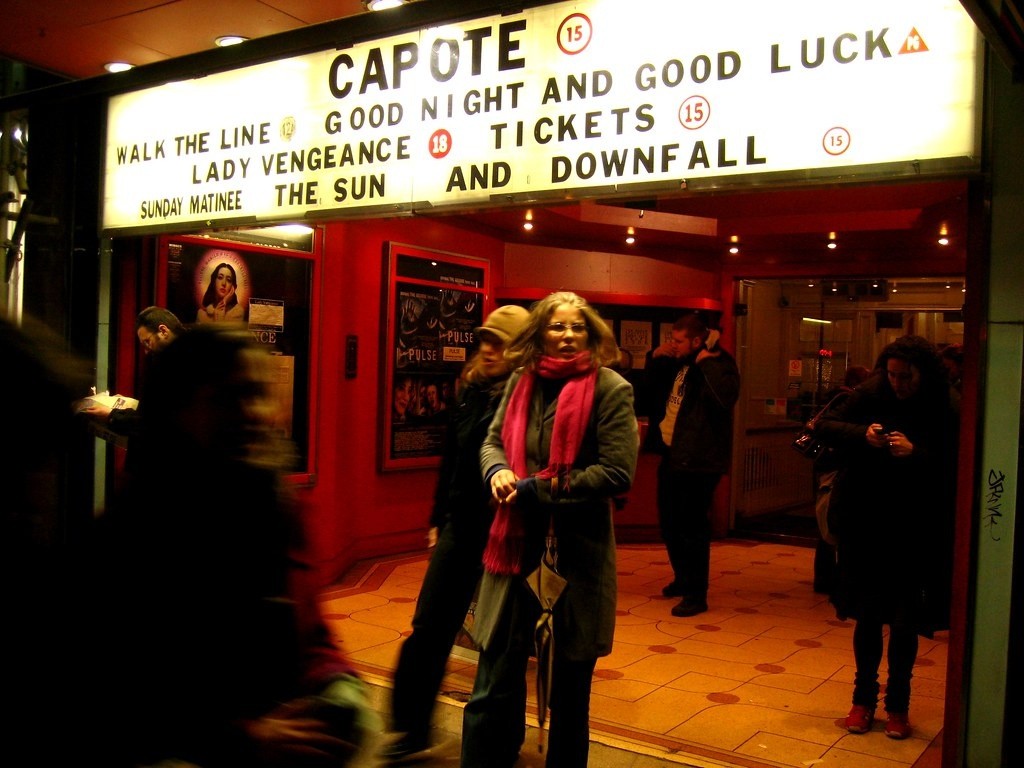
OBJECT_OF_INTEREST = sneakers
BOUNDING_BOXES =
[845,705,875,733]
[885,709,910,738]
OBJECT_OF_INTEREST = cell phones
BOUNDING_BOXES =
[873,427,891,436]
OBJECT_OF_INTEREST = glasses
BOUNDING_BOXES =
[546,323,586,336]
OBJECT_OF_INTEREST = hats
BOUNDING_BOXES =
[474,305,532,344]
[879,334,944,373]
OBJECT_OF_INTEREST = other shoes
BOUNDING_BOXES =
[672,598,708,616]
[662,582,687,597]
[375,733,437,761]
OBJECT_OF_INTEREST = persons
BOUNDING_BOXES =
[0,304,369,768]
[198,264,245,322]
[375,304,534,759]
[816,333,966,738]
[459,292,641,768]
[642,317,741,617]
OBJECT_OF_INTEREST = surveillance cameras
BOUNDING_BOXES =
[780,296,789,305]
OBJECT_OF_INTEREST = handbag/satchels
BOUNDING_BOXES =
[790,392,850,458]
[813,464,850,549]
